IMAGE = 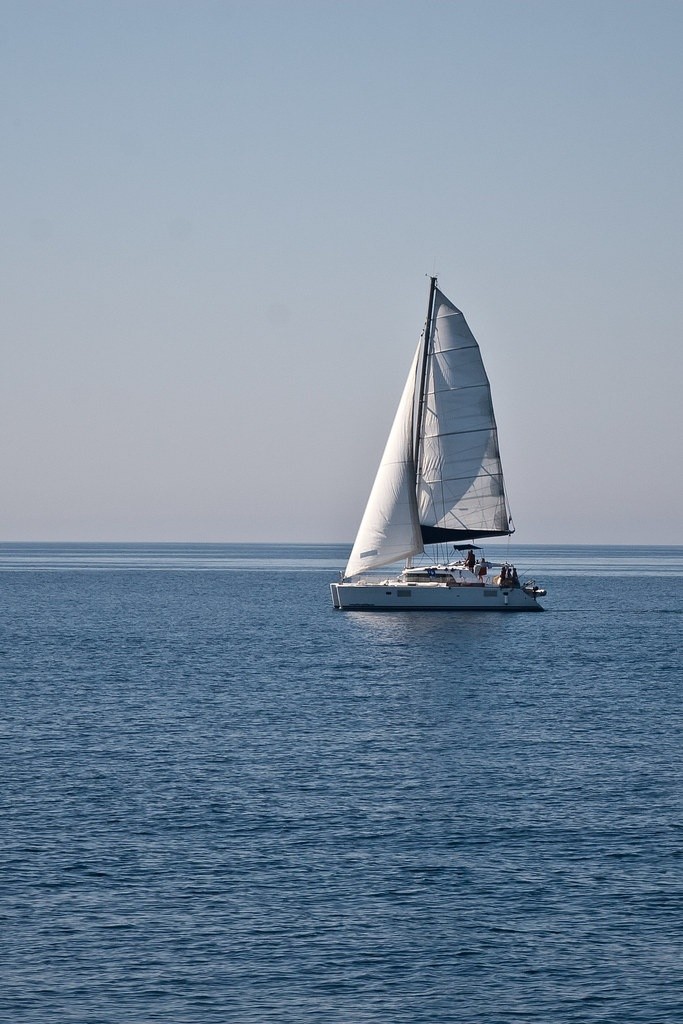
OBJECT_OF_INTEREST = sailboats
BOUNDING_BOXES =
[330,276,547,613]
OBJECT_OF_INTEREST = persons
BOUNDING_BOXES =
[500,566,518,584]
[464,549,487,583]
